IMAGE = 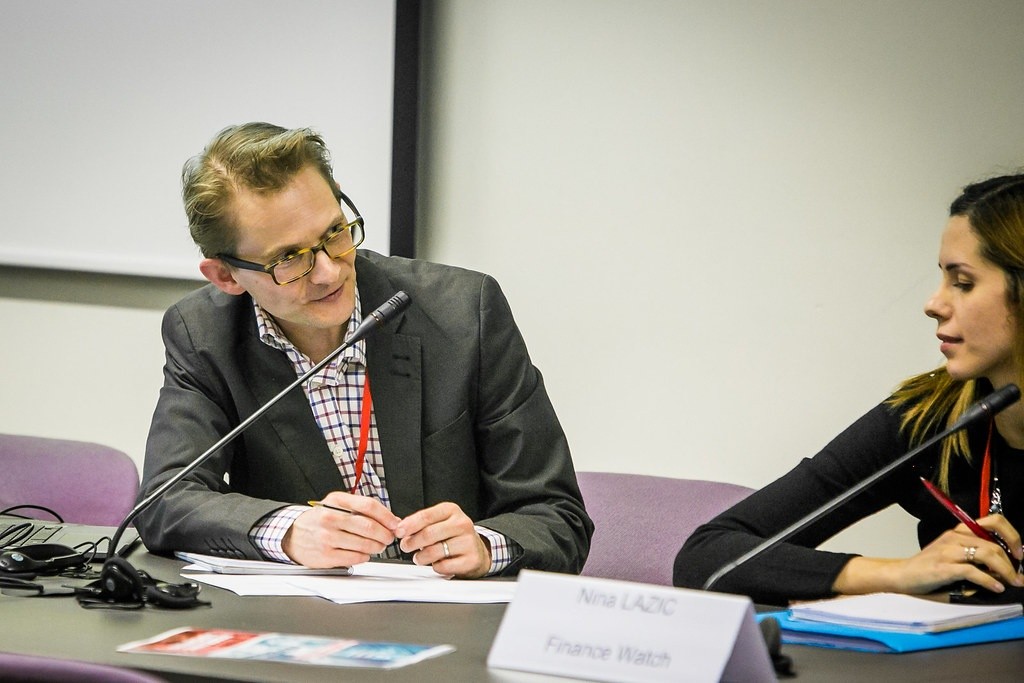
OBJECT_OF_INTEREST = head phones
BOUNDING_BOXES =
[755,616,792,672]
[0,544,86,572]
[101,558,197,609]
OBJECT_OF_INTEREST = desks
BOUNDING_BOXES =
[0,516,1024,683]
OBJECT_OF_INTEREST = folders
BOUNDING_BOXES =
[752,611,1024,654]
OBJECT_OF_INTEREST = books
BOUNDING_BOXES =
[174,550,353,577]
[787,592,1023,635]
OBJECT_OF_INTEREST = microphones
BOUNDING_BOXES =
[100,289,414,597]
[701,383,1023,592]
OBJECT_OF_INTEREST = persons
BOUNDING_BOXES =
[672,174,1024,607]
[132,122,595,580]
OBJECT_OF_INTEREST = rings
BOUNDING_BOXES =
[964,546,977,562]
[441,541,450,558]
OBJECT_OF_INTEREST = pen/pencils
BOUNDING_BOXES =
[920,474,1020,573]
[308,500,360,516]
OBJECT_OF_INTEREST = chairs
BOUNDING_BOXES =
[576,470,759,586]
[0,433,139,531]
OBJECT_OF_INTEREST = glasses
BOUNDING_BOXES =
[217,191,366,286]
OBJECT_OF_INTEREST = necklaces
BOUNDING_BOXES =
[988,423,1024,553]
